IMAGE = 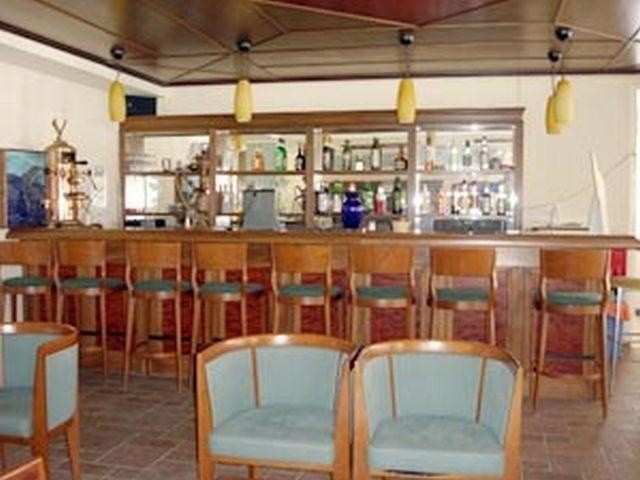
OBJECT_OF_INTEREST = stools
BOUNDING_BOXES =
[190,239,265,388]
[52,237,127,388]
[348,241,417,347]
[124,240,193,391]
[425,247,496,345]
[532,247,611,418]
[612,275,640,387]
[2,237,58,324]
[270,241,342,336]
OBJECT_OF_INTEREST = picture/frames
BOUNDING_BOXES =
[0,149,53,230]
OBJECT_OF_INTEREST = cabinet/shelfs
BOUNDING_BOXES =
[114,104,526,233]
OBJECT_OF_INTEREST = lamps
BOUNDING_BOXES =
[231,39,252,124]
[395,29,418,125]
[107,47,129,124]
[545,50,561,134]
[553,27,572,121]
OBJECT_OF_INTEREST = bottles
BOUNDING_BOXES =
[319,132,410,231]
[160,136,304,219]
[424,133,506,219]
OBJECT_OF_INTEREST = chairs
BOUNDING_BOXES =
[195,333,360,479]
[352,339,525,480]
[0,323,80,480]
